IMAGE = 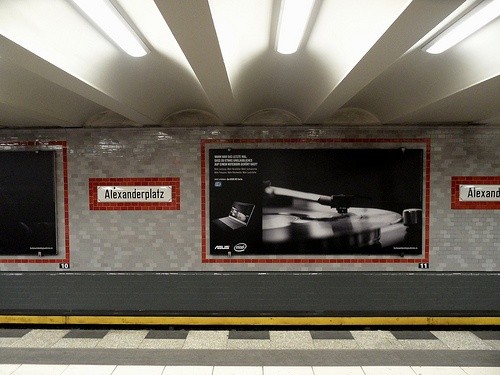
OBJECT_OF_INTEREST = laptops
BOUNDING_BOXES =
[212,202,255,233]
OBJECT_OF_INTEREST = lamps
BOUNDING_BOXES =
[418,0,500,56]
[268,0,324,54]
[66,0,153,60]
